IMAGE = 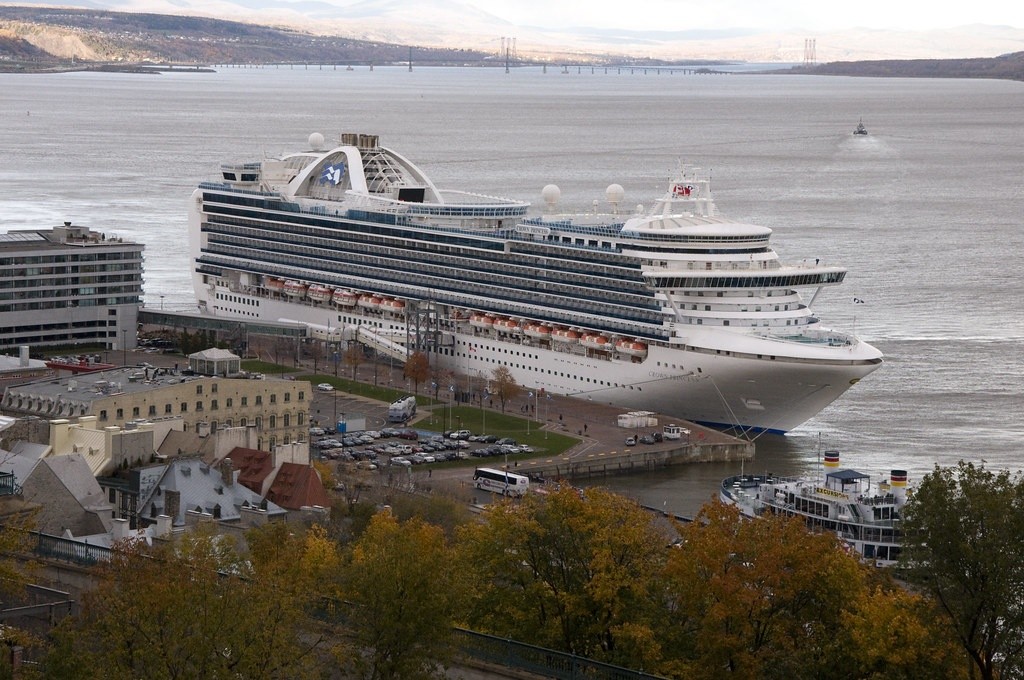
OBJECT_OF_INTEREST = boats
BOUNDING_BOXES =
[522,324,550,339]
[549,330,578,343]
[331,288,357,307]
[264,278,284,294]
[577,333,608,350]
[304,285,331,302]
[615,341,647,357]
[492,319,517,335]
[469,314,494,329]
[720,430,945,583]
[380,300,404,314]
[355,296,381,310]
[282,282,305,298]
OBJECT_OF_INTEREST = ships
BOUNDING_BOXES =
[188,128,888,432]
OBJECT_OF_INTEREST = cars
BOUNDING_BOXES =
[307,419,533,470]
[318,383,334,391]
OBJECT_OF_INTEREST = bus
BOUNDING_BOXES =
[472,468,531,500]
[387,394,416,424]
